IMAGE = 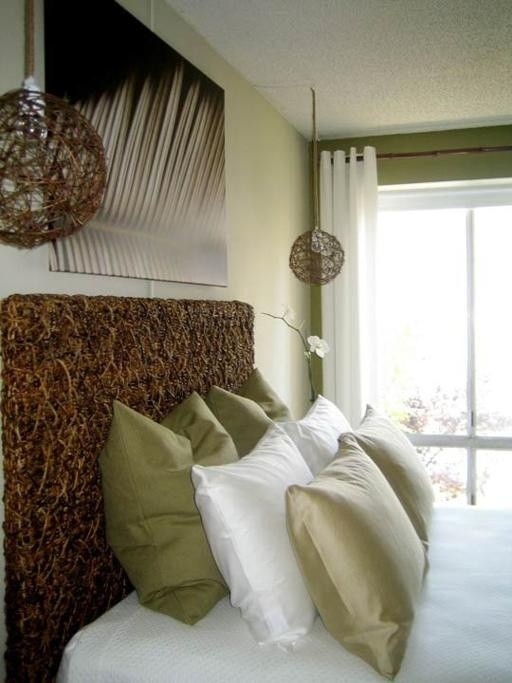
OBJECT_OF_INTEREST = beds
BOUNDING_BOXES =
[0,291,512,683]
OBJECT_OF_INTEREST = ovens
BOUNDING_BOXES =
[0,291,512,683]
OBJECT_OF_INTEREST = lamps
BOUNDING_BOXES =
[288,87,345,284]
[0,0,109,250]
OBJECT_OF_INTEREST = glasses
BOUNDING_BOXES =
[0,0,109,250]
[288,87,345,284]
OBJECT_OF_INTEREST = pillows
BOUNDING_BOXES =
[206,370,291,460]
[191,422,317,654]
[277,394,354,477]
[286,434,425,679]
[349,404,437,552]
[100,390,238,625]
